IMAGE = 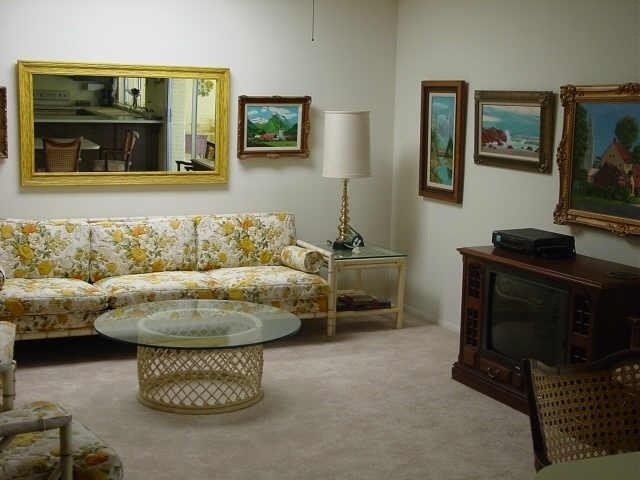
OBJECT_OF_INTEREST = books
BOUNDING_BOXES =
[345,290,374,303]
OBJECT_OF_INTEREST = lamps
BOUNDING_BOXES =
[322,110,374,253]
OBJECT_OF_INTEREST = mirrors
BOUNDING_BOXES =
[15,57,231,188]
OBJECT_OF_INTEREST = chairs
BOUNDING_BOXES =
[173,141,215,170]
[89,129,141,172]
[41,136,85,171]
[519,349,640,464]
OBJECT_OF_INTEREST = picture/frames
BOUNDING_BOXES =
[236,94,313,160]
[473,89,554,176]
[552,80,640,238]
[414,79,472,206]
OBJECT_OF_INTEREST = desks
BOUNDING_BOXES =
[534,449,640,479]
[33,134,100,154]
[190,158,215,171]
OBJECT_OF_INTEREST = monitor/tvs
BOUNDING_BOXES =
[479,266,572,373]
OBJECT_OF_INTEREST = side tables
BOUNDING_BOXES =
[322,246,410,337]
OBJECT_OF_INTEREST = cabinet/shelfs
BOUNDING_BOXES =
[450,240,639,451]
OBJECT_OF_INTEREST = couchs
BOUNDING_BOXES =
[0,316,125,479]
[0,211,335,357]
